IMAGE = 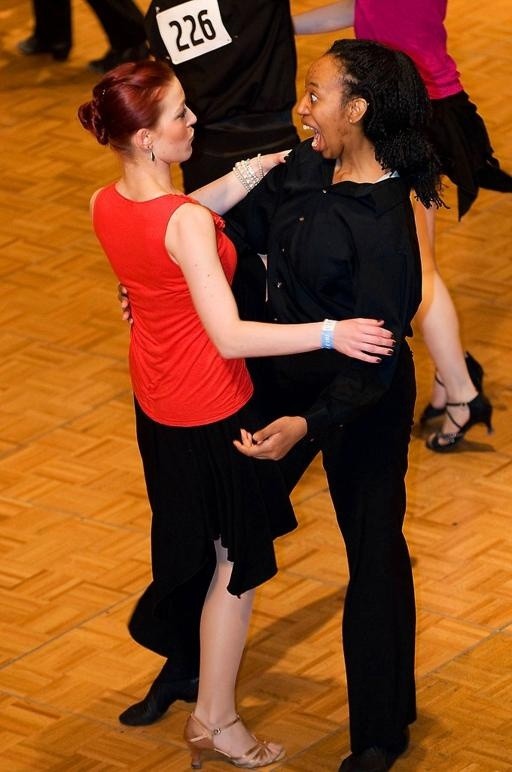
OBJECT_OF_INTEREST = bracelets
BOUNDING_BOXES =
[232,159,260,192]
[319,319,336,350]
[257,154,265,179]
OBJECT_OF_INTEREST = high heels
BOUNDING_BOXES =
[425,392,495,451]
[420,350,483,424]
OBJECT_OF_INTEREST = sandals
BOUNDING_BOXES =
[183,711,286,769]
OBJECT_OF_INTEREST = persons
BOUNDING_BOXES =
[137,2,297,222]
[292,0,512,452]
[76,62,396,769]
[120,40,451,772]
[19,0,151,73]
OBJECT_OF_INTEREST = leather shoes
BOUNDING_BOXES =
[119,675,199,726]
[338,724,410,772]
[19,38,71,61]
[89,42,149,73]
